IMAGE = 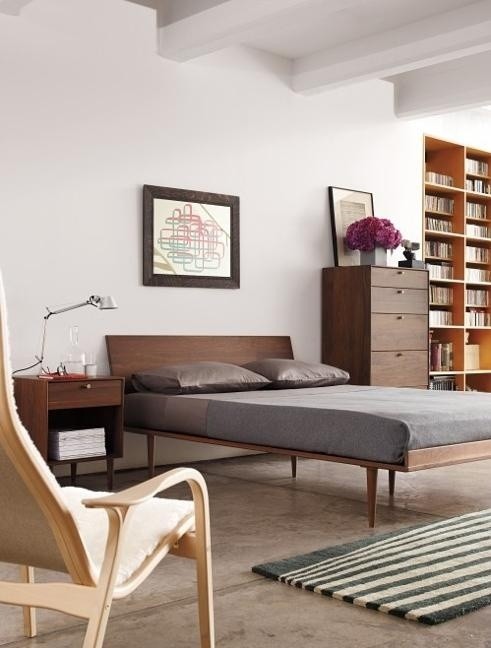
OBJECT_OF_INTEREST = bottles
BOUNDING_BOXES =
[66,325,84,375]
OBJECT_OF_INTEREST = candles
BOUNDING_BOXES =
[82,365,96,379]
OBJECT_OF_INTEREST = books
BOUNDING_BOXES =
[40,373,87,379]
[425,158,491,392]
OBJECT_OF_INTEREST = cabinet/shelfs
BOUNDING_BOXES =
[322,264,429,391]
[420,135,489,394]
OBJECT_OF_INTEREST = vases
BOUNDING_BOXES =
[359,243,388,265]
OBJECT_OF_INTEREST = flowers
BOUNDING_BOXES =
[345,215,403,251]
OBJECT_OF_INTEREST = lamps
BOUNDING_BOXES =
[13,292,118,377]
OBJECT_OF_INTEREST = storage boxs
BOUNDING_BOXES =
[465,343,480,369]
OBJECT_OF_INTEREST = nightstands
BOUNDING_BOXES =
[14,375,125,490]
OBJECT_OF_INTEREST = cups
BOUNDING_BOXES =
[83,352,97,378]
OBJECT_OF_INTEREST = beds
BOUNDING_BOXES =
[106,334,489,527]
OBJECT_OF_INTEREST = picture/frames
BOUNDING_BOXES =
[141,186,240,289]
[328,186,376,268]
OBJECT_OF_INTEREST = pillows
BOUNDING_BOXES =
[129,362,272,394]
[238,358,349,388]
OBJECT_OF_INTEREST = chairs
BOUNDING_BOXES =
[1,284,216,648]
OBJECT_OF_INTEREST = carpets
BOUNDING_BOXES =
[252,507,489,624]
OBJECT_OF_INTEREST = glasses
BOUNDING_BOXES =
[41,364,68,376]
